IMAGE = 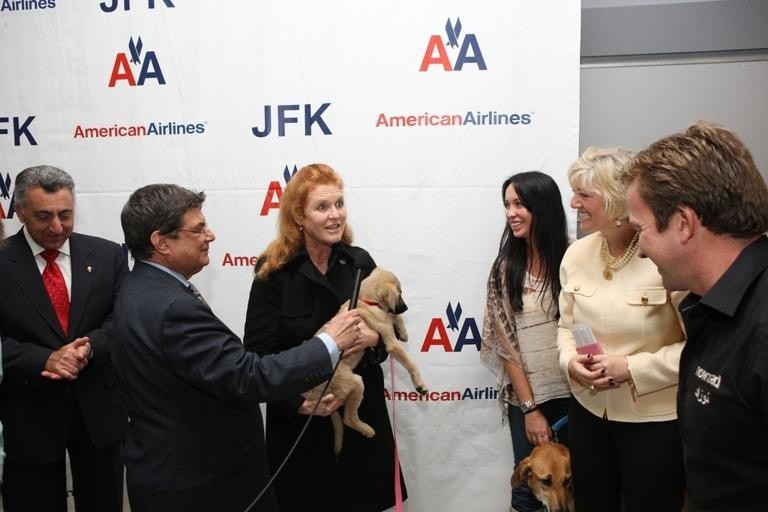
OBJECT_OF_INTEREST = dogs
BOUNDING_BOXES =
[301,266,429,455]
[509,439,575,512]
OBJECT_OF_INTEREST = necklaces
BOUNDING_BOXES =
[601,232,640,283]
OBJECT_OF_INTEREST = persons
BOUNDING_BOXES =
[115,182,362,512]
[243,163,410,511]
[618,121,766,512]
[480,170,580,511]
[0,164,132,512]
[555,146,694,512]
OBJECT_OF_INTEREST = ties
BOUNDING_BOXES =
[188,280,208,305]
[40,250,69,336]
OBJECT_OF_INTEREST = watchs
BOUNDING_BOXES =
[520,400,534,412]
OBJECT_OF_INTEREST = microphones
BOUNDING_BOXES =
[347,249,370,309]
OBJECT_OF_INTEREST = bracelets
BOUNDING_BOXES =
[624,356,635,394]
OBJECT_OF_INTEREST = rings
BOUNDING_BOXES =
[588,386,594,393]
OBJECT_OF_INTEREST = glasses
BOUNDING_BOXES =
[171,226,207,234]
